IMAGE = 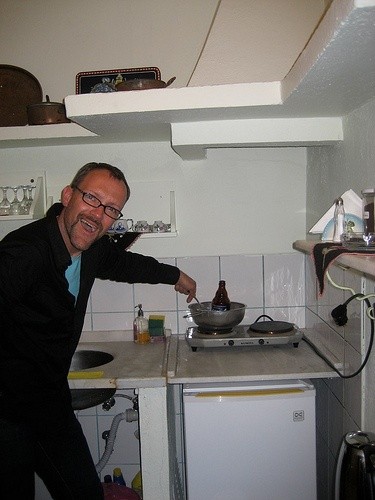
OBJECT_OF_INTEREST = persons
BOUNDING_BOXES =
[0,162,196,500]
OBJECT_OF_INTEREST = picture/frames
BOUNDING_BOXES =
[75,66,161,95]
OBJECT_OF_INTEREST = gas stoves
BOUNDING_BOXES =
[185,321,303,351]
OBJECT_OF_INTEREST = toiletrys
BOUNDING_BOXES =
[133,304,150,345]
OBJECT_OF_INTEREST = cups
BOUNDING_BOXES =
[361,188,375,235]
[152,221,165,232]
[114,218,133,233]
[135,221,150,233]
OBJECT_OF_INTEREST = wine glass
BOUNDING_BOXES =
[10,186,20,215]
[19,185,30,214]
[24,186,35,214]
[0,186,10,215]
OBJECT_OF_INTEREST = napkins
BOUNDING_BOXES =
[309,189,366,234]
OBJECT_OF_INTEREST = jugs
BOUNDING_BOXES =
[334,431,375,500]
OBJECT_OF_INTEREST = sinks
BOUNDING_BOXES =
[68,349,117,410]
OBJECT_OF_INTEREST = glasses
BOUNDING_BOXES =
[74,186,124,221]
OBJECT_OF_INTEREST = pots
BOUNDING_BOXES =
[105,76,176,89]
[27,96,68,125]
[183,301,246,329]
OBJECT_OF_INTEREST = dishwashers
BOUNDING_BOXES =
[183,380,317,500]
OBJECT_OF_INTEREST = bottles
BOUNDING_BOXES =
[211,280,231,311]
[333,198,344,242]
